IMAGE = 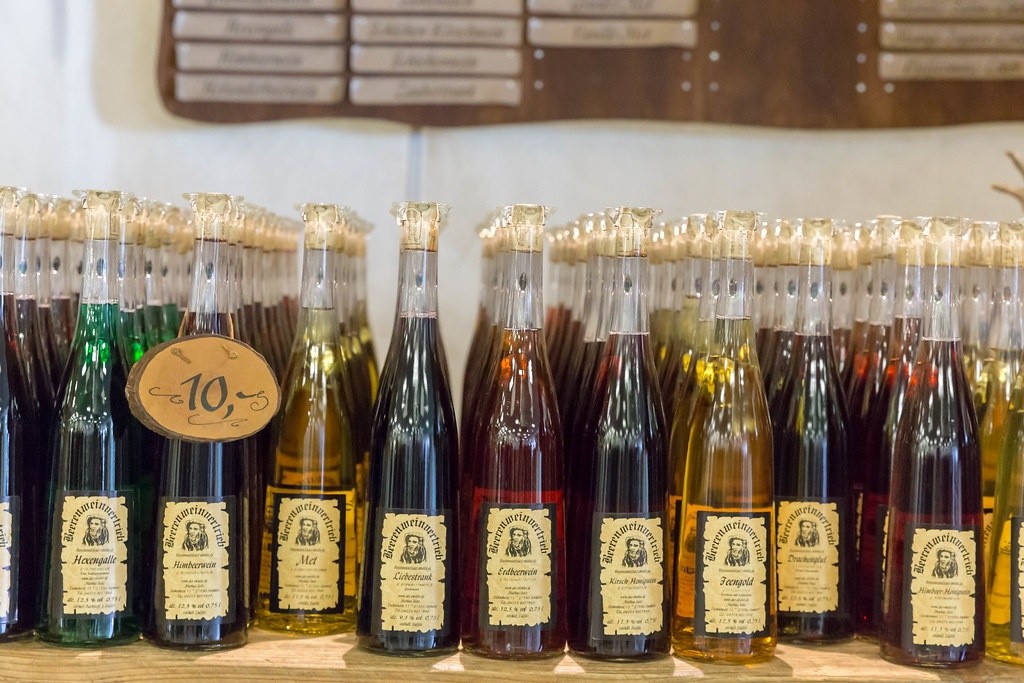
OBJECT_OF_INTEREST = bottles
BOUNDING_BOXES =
[1,184,1024,667]
